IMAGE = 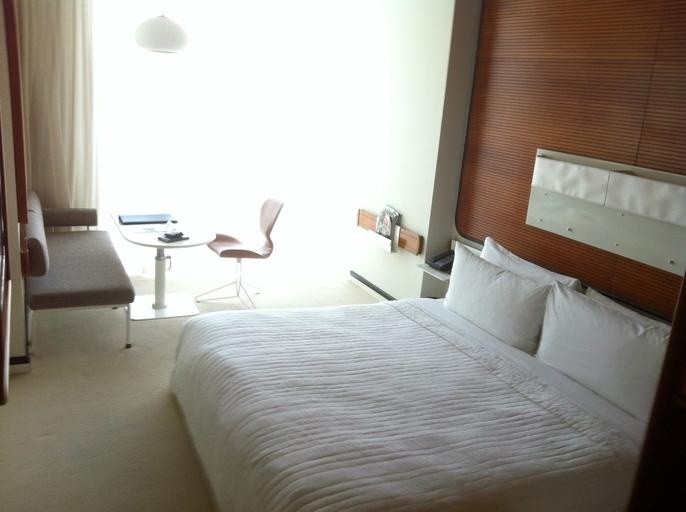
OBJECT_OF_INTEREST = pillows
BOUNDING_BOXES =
[537,279,670,423]
[446,241,552,354]
[481,237,582,288]
[585,287,672,331]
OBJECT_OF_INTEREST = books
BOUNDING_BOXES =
[372,205,399,237]
[117,214,176,225]
[157,236,188,244]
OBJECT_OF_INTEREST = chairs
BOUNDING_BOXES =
[196,199,283,308]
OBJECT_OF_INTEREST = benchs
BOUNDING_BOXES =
[26,190,134,348]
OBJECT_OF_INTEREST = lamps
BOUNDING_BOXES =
[135,13,186,53]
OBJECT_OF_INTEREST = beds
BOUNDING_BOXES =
[168,297,649,511]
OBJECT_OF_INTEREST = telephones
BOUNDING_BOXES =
[426,250,454,272]
